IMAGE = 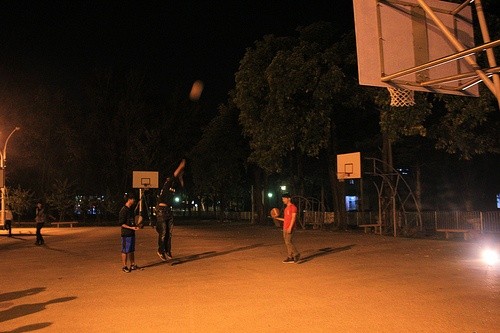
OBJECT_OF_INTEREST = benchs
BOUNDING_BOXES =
[51,221,78,228]
[436,228,473,241]
[358,224,384,233]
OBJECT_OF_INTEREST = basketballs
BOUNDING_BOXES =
[188,79,204,100]
[270,207,280,217]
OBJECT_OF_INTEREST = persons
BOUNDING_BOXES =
[34,203,56,245]
[276,193,300,264]
[118,194,140,273]
[5,205,13,236]
[155,158,185,262]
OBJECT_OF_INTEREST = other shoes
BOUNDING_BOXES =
[156,251,167,261]
[36,240,44,246]
[130,263,141,270]
[282,257,294,263]
[165,251,172,259]
[122,265,130,273]
[294,253,301,264]
